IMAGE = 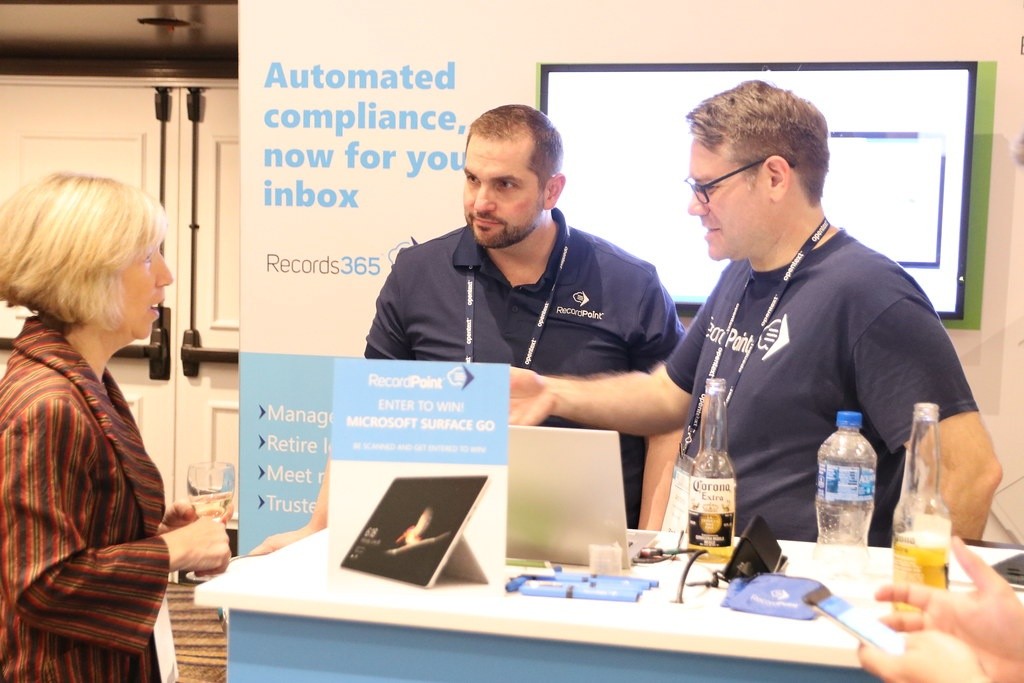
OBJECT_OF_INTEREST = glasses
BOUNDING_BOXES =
[684,153,796,204]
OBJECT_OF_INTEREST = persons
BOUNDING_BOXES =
[363,104,690,535]
[0,175,234,683]
[509,81,1007,546]
[855,533,1024,683]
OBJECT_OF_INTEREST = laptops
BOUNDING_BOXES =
[504,424,659,569]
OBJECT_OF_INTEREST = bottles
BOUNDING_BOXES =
[688,378,736,562]
[892,401,952,611]
[816,410,877,599]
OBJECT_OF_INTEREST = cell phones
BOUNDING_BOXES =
[804,588,905,657]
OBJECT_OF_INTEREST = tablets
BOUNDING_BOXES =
[339,474,491,591]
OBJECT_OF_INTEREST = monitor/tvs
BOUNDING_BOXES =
[536,61,977,318]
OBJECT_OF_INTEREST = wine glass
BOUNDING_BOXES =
[187,463,235,581]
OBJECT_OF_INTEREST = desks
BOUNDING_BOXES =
[193,527,1024,683]
